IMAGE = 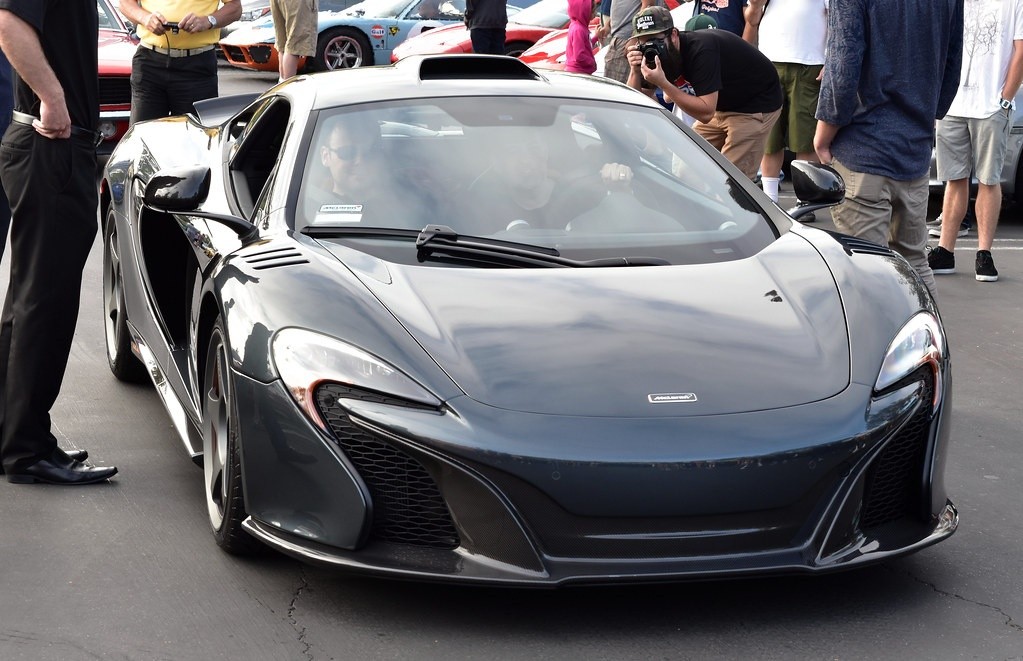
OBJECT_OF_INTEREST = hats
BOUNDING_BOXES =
[627,5,673,37]
[686,13,718,31]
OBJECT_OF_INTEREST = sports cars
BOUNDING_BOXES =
[95,50,961,592]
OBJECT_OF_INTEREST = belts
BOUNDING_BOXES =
[141,39,215,57]
[12,110,103,148]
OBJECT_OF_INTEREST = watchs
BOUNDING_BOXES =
[999,99,1012,111]
[207,15,217,31]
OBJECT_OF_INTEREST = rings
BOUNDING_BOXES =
[619,173,625,177]
[191,24,194,28]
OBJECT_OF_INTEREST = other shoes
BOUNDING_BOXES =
[795,201,815,222]
[755,179,781,191]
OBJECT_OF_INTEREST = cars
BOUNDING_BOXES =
[391,0,683,69]
[305,0,537,75]
[218,1,364,75]
[97,0,145,156]
[517,0,689,69]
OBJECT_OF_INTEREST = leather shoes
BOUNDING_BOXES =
[7,452,118,484]
[63,449,88,462]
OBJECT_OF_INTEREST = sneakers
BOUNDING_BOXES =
[929,221,968,238]
[926,212,944,230]
[925,244,955,274]
[975,249,998,281]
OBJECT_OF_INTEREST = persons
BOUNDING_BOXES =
[270,0,318,85]
[464,0,508,56]
[471,130,636,232]
[2,0,118,486]
[565,1,1023,282]
[299,112,430,240]
[120,0,243,131]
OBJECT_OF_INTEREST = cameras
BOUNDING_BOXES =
[163,22,181,35]
[634,37,668,64]
